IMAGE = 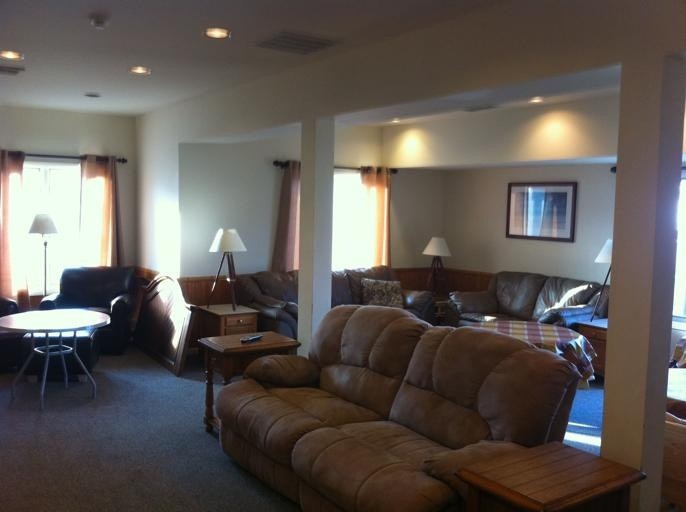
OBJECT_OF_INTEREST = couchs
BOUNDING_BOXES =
[237,264,437,339]
[213,304,581,511]
[20,326,99,375]
[449,272,609,328]
[0,296,20,368]
[38,266,137,355]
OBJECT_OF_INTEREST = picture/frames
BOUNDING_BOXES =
[132,274,199,377]
[505,181,578,243]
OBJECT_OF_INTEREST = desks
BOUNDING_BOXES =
[468,320,598,393]
[0,307,110,411]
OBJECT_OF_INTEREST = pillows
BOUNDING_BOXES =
[360,276,405,310]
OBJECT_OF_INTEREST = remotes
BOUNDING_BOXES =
[240,333,263,343]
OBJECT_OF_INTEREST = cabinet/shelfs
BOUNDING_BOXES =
[577,317,607,379]
[198,331,301,432]
[458,441,649,512]
[197,304,259,336]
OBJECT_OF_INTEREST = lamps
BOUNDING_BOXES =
[591,238,613,323]
[208,228,246,311]
[29,213,59,292]
[422,237,452,288]
